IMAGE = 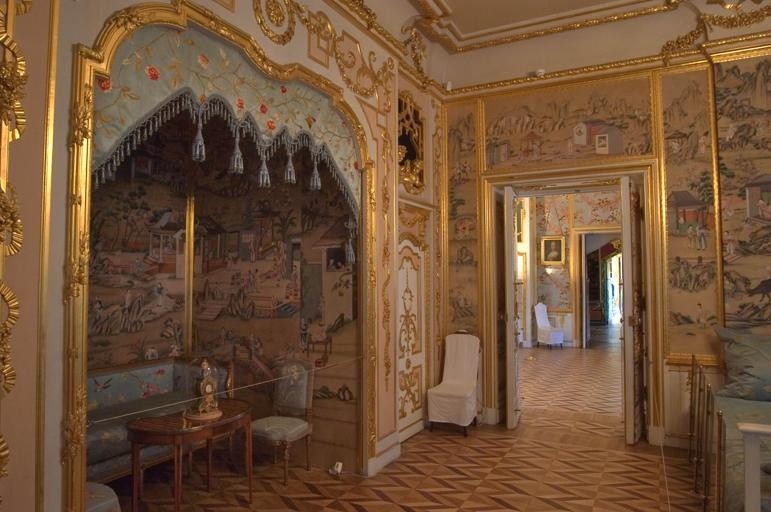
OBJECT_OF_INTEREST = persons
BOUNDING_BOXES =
[546,240,560,260]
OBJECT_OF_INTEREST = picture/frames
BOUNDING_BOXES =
[540,234,566,265]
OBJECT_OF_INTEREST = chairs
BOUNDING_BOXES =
[82,478,124,512]
[535,301,565,351]
[250,358,316,486]
[426,333,481,437]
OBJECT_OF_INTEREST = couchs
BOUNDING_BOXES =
[704,325,771,511]
[83,357,235,486]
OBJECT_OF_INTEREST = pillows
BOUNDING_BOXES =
[713,325,771,402]
